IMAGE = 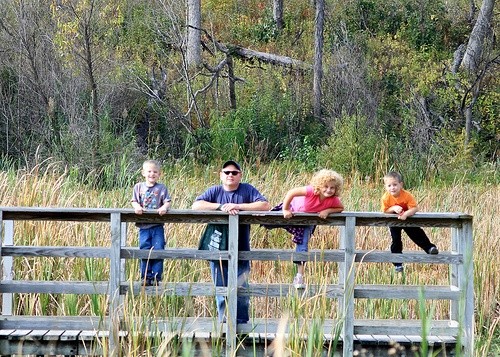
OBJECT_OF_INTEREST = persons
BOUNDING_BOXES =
[258,169,345,290]
[381,171,438,272]
[192,160,270,324]
[131,160,171,286]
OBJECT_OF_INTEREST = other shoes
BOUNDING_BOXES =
[221,332,250,338]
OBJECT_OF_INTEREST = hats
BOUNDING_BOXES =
[222,161,240,170]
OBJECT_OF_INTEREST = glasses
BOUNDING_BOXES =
[222,171,241,176]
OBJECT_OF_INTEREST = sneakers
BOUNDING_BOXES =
[293,273,305,289]
[141,277,150,286]
[430,247,439,255]
[150,277,159,286]
[396,264,403,271]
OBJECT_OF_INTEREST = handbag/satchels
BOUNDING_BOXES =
[199,223,227,263]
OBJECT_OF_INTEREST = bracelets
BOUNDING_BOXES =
[215,204,223,210]
